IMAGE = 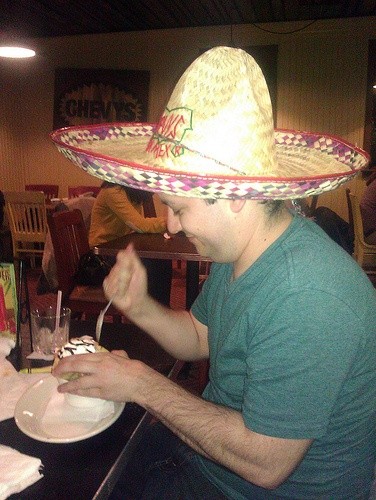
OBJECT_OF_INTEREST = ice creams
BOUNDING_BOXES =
[52,335,112,409]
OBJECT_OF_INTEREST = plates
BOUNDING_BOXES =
[13,374,126,444]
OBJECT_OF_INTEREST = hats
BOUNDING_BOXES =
[49,46,371,201]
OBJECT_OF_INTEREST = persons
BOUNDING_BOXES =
[361,171,376,244]
[89,180,172,306]
[285,198,309,218]
[49,46,376,500]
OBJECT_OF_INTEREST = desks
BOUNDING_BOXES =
[0,319,185,500]
[93,231,212,311]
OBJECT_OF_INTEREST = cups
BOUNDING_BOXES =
[56,376,106,408]
[30,307,70,357]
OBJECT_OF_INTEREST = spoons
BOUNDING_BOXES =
[49,290,64,355]
[95,299,112,342]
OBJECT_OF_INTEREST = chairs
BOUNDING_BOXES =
[348,192,376,275]
[2,184,125,324]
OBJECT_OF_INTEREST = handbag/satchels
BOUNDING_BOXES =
[75,251,108,287]
